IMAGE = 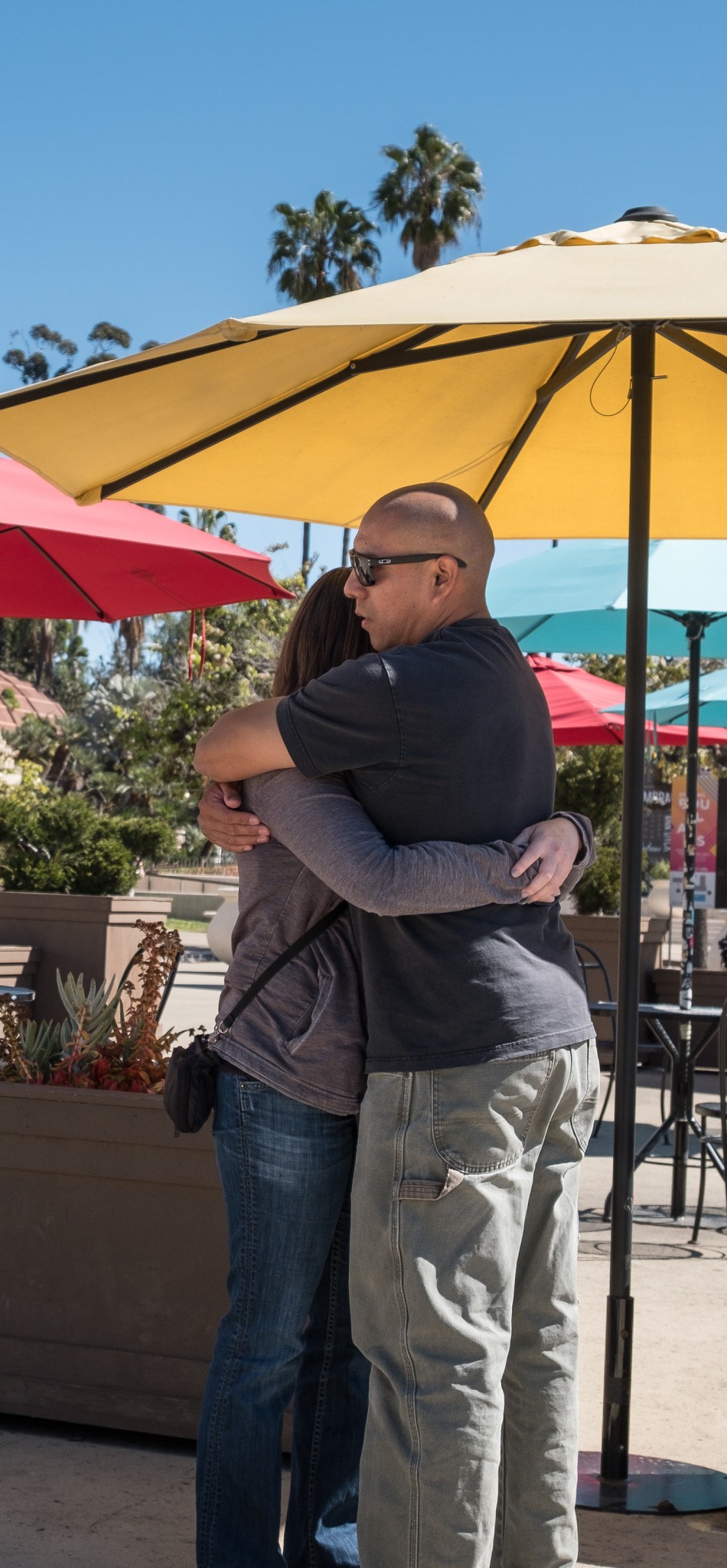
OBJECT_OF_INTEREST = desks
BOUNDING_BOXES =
[587,1001,727,1225]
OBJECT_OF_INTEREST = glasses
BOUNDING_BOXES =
[351,550,469,588]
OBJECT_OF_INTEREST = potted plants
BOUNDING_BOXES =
[0,923,297,1462]
[0,777,179,1038]
[561,817,672,1042]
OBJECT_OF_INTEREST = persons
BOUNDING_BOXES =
[191,480,601,1568]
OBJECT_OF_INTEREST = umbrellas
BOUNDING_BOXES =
[0,205,727,1512]
[0,444,303,622]
[476,540,727,1223]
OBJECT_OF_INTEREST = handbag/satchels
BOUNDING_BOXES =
[161,1034,222,1136]
